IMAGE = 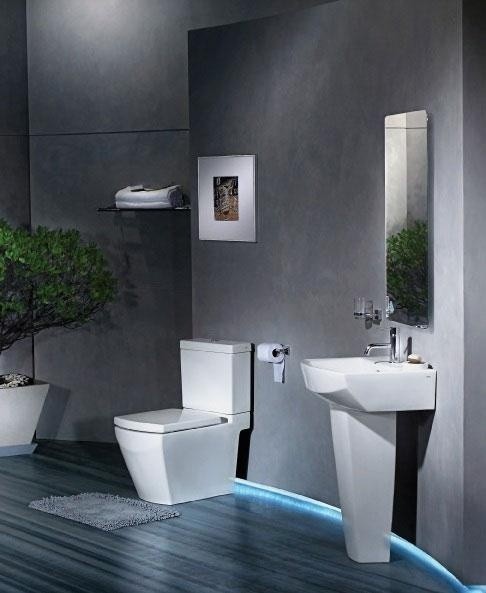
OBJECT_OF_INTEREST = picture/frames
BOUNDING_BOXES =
[196,153,258,243]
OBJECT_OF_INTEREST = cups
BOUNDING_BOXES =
[353,296,368,318]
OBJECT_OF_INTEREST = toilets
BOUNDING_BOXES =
[114,338,253,506]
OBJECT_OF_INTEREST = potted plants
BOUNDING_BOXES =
[0,213,120,456]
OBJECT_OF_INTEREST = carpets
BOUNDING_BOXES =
[28,489,181,532]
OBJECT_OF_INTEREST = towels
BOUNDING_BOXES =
[114,183,184,208]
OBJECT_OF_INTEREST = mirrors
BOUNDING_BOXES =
[381,107,433,329]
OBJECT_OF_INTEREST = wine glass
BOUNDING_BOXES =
[216,205,231,220]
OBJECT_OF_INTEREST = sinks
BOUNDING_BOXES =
[299,357,437,414]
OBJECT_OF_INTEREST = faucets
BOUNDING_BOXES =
[364,327,397,364]
